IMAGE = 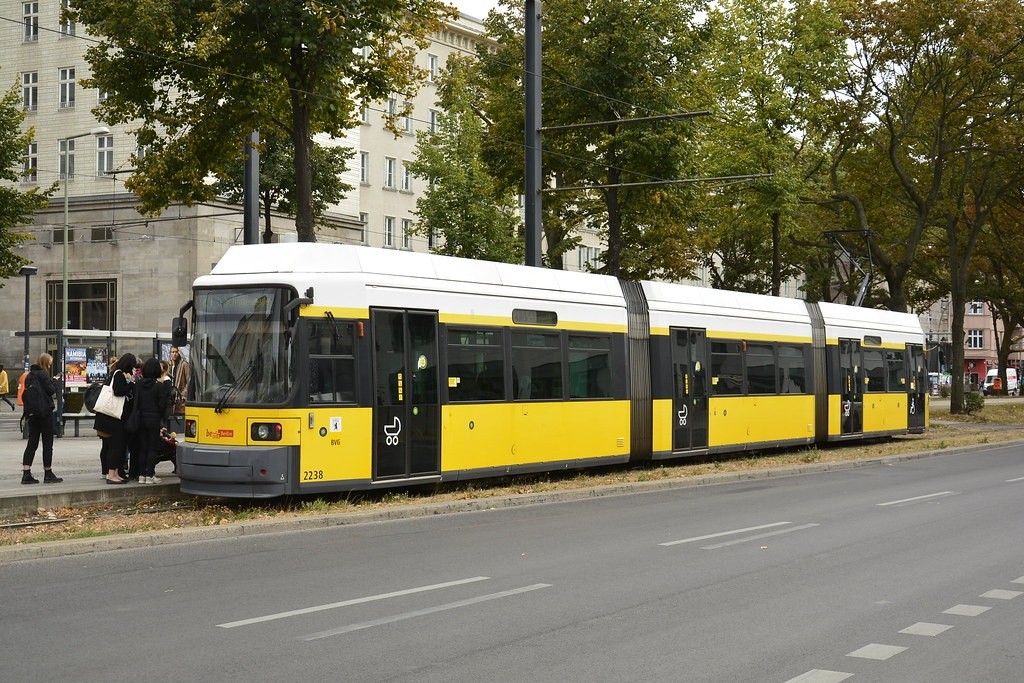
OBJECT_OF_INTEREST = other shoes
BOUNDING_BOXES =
[12,404,15,411]
[120,478,128,482]
[106,478,126,484]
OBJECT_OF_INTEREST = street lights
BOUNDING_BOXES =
[61,126,110,329]
[17,265,38,372]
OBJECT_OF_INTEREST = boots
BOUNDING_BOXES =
[44,469,63,484]
[21,469,39,484]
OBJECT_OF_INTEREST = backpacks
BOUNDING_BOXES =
[23,372,54,418]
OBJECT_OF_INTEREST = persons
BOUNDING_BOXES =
[61,393,84,435]
[93,345,190,485]
[88,349,103,363]
[17,354,60,484]
[0,365,15,411]
[352,339,393,464]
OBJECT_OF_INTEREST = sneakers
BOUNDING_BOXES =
[139,476,146,484]
[146,476,161,484]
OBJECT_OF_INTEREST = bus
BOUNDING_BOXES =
[168,242,930,509]
[928,372,943,396]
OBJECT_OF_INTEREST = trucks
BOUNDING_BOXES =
[983,369,1018,396]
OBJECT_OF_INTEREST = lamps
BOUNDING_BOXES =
[42,228,152,249]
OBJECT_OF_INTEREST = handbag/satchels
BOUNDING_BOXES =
[84,383,102,413]
[94,369,125,419]
[122,409,140,434]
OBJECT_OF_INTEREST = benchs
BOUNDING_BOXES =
[62,412,96,437]
